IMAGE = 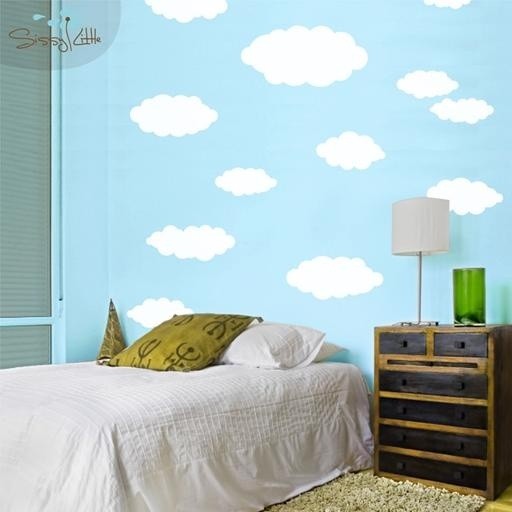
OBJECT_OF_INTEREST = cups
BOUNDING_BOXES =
[452,268,486,328]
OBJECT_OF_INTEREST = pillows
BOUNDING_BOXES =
[105,311,341,371]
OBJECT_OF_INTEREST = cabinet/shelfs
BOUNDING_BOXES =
[372,325,511,501]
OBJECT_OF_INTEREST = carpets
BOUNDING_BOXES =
[0,361,363,512]
[267,470,485,512]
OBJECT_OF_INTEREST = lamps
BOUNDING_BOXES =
[389,197,449,324]
